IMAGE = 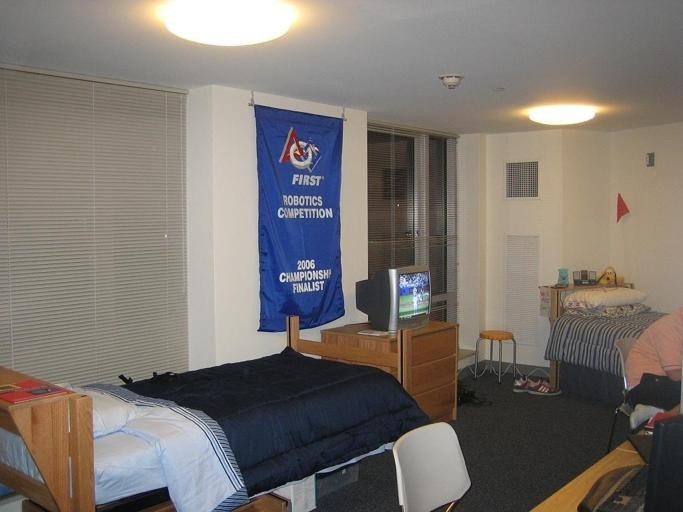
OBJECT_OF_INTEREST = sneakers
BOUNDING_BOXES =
[513,375,563,397]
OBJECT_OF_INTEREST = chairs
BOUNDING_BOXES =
[388,420,471,512]
[606,335,640,454]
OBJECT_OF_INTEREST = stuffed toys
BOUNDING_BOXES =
[595,265,616,286]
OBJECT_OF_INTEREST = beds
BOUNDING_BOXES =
[546,282,671,409]
[1,347,430,512]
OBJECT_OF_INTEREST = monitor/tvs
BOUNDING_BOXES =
[356,264,432,333]
[644,413,683,512]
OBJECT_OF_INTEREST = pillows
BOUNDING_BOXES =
[564,287,651,320]
[52,382,127,440]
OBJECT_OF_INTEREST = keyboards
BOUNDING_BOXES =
[577,464,648,512]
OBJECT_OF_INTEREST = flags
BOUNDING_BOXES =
[253,102,345,335]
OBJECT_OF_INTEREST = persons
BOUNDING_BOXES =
[622,305,682,429]
[399,271,428,315]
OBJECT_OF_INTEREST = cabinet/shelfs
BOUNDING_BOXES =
[319,320,458,427]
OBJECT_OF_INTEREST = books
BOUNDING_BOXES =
[0,377,68,404]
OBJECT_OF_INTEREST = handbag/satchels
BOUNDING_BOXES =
[457,387,493,407]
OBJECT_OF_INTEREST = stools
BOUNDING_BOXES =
[471,328,516,385]
